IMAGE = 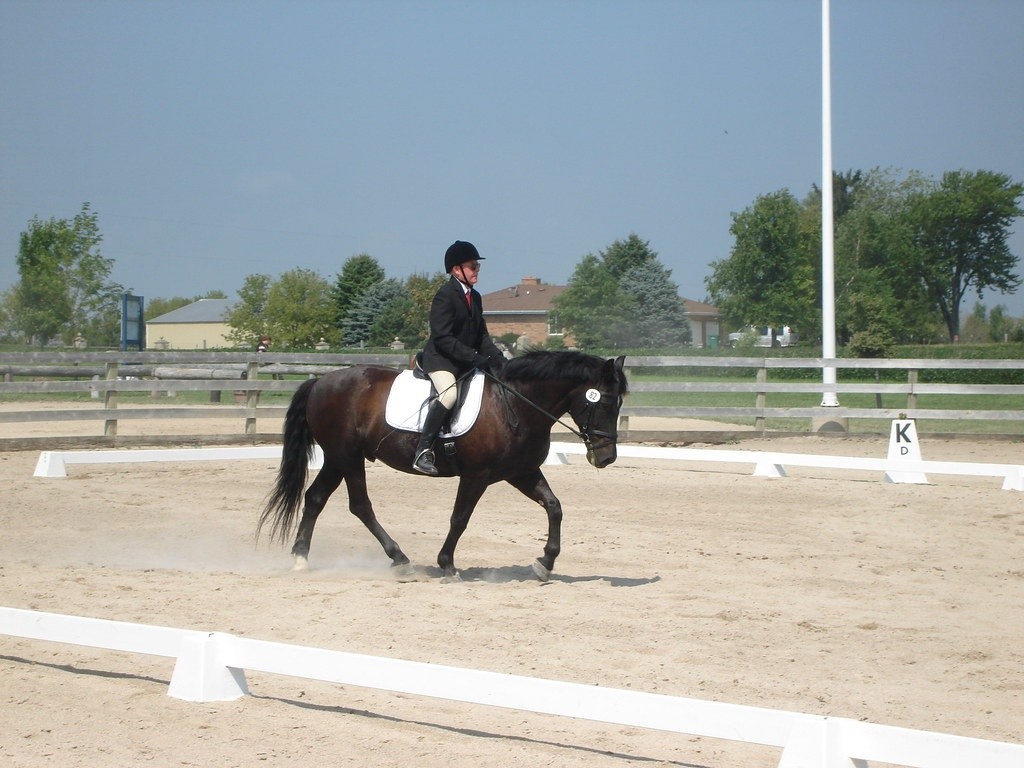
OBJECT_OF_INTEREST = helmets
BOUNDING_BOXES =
[444,240,486,274]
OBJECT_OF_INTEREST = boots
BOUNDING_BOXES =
[413,400,450,477]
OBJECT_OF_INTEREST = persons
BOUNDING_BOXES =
[413,241,506,475]
[256,336,284,380]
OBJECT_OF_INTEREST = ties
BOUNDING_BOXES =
[464,290,472,313]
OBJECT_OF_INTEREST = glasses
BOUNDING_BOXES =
[463,261,482,271]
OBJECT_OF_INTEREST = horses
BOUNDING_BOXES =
[253,340,629,585]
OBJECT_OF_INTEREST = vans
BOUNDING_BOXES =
[729,313,800,348]
